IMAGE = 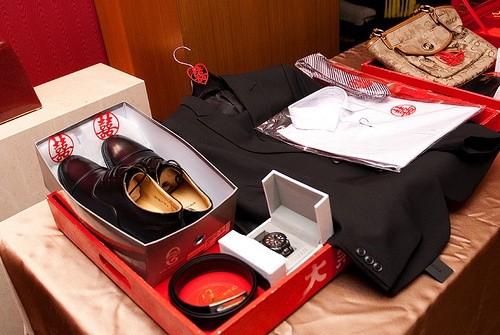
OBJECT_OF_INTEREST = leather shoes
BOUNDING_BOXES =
[59,154,183,243]
[101,135,212,225]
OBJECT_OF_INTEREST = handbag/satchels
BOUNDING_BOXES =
[366,4,497,89]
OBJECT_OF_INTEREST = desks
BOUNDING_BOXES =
[0,62,153,223]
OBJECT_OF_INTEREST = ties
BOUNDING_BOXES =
[295,53,392,103]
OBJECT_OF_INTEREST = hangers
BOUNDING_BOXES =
[172,45,224,99]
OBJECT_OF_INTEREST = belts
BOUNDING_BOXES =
[168,251,271,330]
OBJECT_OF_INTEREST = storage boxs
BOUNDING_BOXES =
[0,41,500,335]
[34,100,240,289]
[45,169,347,334]
